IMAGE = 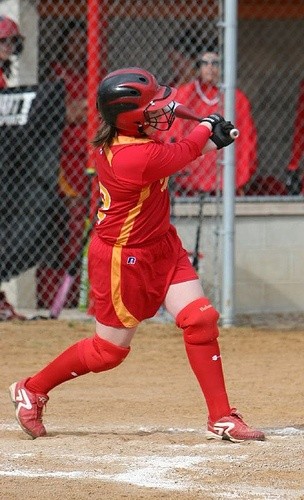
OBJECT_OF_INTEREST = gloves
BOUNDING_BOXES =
[209,120,235,150]
[285,167,303,194]
[199,112,225,132]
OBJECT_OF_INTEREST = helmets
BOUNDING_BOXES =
[96,67,178,136]
[0,18,19,39]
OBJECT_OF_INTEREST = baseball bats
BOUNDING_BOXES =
[163,101,240,139]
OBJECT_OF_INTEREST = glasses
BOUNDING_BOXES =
[199,60,221,68]
[0,36,19,44]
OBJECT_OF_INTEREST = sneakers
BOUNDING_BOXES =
[8,375,49,438]
[207,407,266,442]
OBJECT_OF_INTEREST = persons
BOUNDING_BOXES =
[7,67,265,443]
[0,16,26,94]
[160,48,258,196]
[37,19,92,310]
